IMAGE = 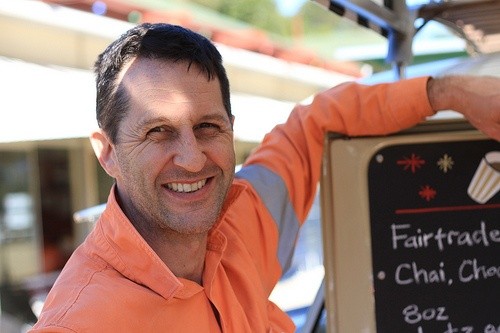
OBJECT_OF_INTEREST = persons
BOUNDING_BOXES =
[25,22,499,333]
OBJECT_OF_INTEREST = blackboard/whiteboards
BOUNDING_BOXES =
[324,118,500,333]
